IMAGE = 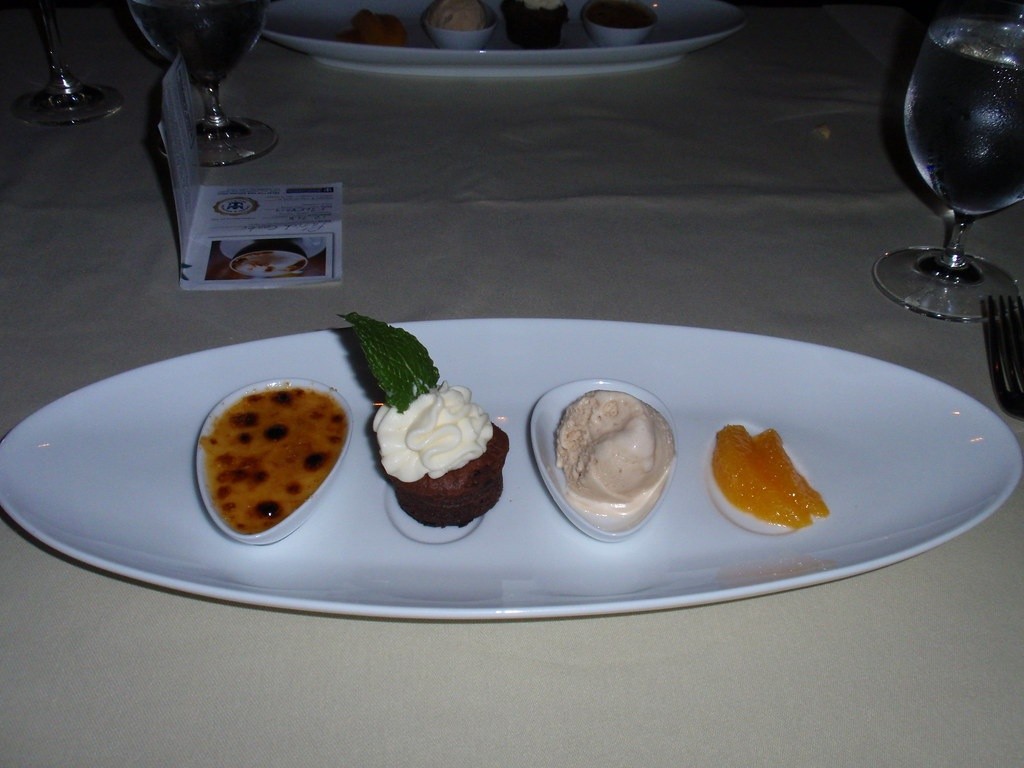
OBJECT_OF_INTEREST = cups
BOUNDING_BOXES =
[229,240,309,277]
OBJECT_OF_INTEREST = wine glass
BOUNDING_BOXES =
[9,0,125,127]
[127,0,280,166]
[871,0,1024,323]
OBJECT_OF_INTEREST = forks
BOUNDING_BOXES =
[988,294,1022,422]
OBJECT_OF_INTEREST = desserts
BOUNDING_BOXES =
[338,310,510,530]
[201,387,348,536]
[555,391,675,514]
[352,0,657,46]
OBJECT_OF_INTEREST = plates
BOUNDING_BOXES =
[220,239,326,259]
[0,319,1023,620]
[260,0,750,76]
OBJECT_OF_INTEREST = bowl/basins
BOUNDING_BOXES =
[580,0,658,48]
[529,378,677,544]
[423,4,499,50]
[196,378,353,546]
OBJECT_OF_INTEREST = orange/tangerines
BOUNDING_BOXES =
[713,421,831,529]
